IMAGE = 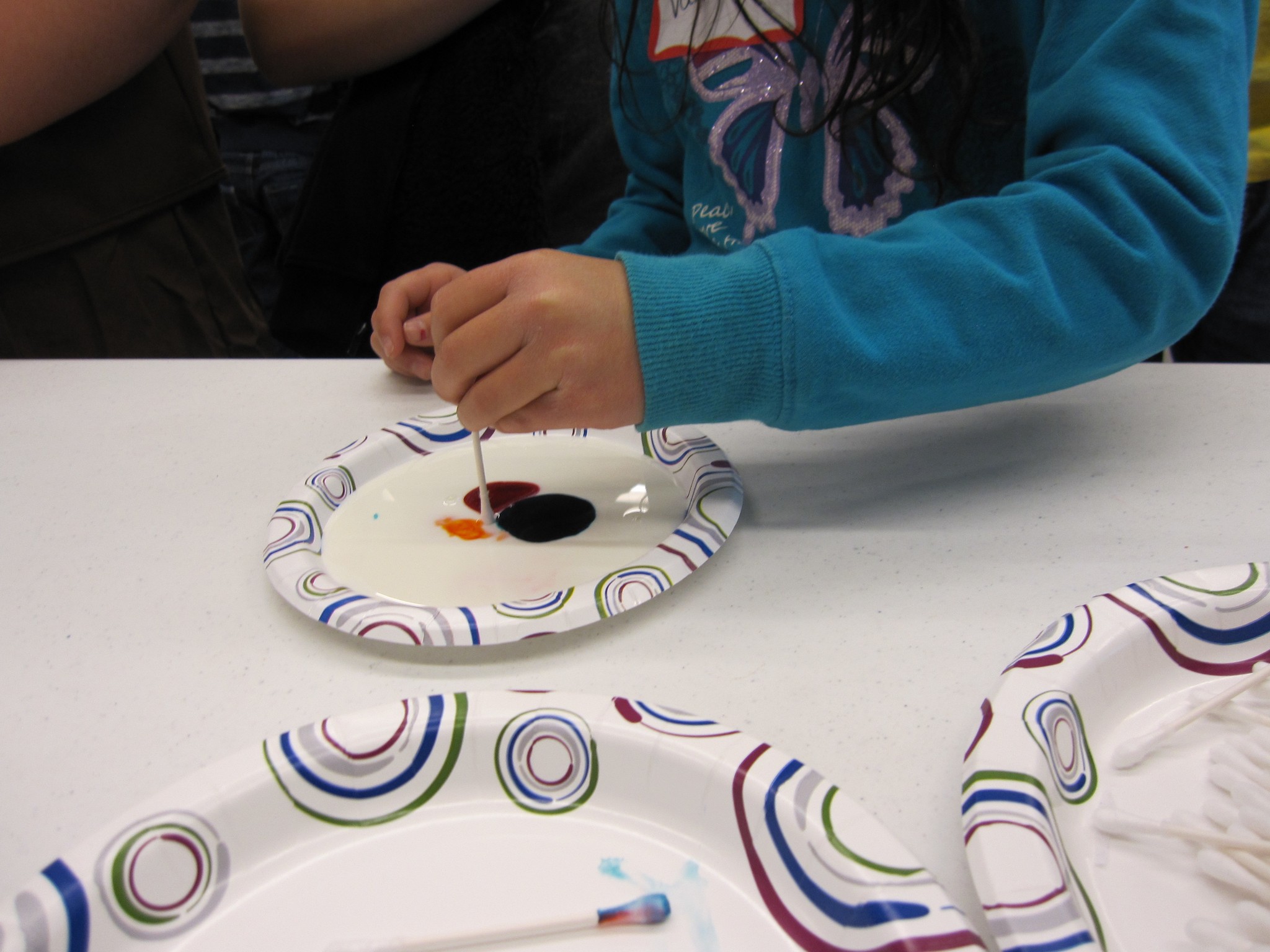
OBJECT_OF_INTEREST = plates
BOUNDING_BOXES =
[963,555,1269,952]
[262,399,745,645]
[0,687,986,952]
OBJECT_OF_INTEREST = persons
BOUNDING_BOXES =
[369,0,1259,435]
[0,1,482,359]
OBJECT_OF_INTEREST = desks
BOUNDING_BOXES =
[1,356,1269,952]
